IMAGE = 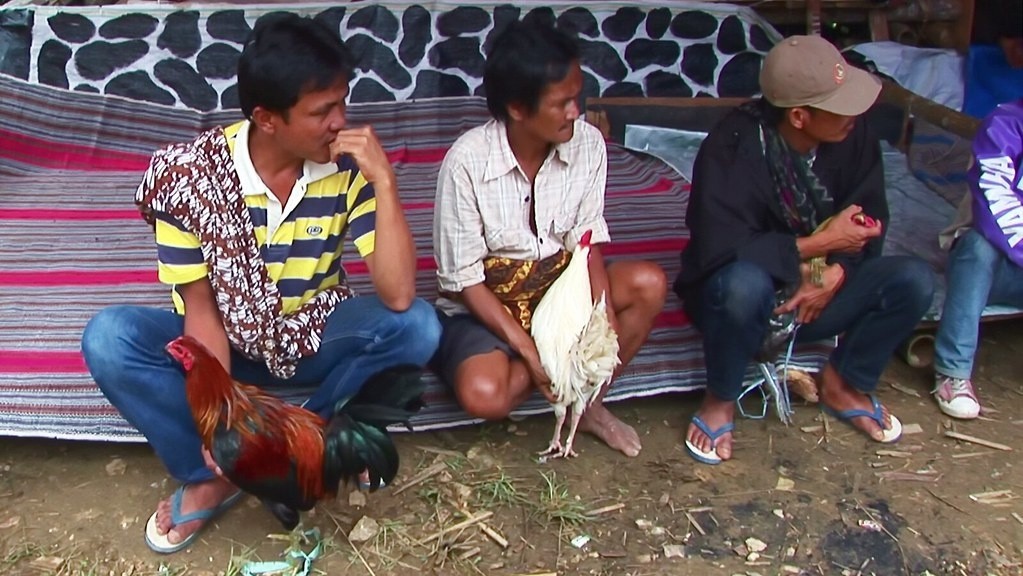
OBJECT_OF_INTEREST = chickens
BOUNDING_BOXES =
[165,336,430,556]
[530,231,622,458]
[761,210,876,361]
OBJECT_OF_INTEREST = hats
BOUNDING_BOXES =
[758,35,883,116]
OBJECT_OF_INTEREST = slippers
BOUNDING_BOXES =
[817,393,902,443]
[684,416,735,465]
[144,482,244,554]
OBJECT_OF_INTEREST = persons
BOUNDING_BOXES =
[932,101,1023,421]
[427,21,669,457]
[83,11,442,553]
[673,35,937,465]
[991,0,1023,67]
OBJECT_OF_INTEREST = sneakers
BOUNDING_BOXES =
[930,370,981,419]
